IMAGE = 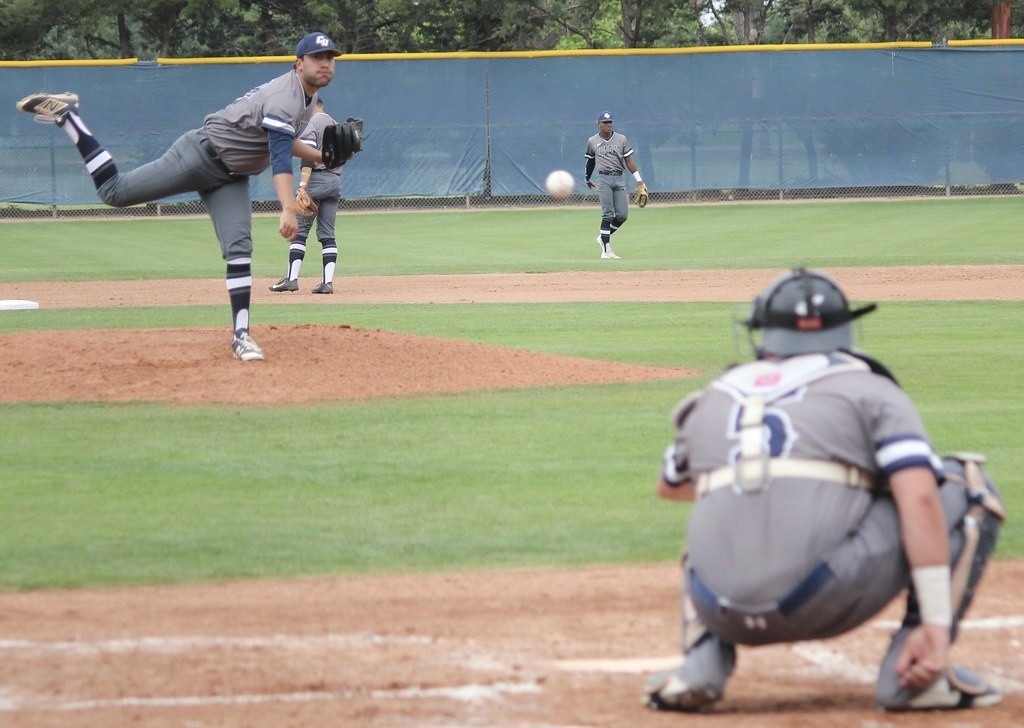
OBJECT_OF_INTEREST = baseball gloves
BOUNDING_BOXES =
[632,183,648,209]
[294,191,318,217]
[322,116,364,171]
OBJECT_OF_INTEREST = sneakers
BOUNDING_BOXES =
[231,335,264,361]
[269,277,298,292]
[311,281,334,294]
[18,92,80,128]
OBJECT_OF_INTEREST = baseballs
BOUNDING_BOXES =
[545,170,575,199]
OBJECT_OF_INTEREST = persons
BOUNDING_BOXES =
[643,267,1004,711]
[584,111,648,258]
[14,32,356,360]
[268,96,347,293]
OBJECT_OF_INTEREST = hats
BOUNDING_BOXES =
[598,111,614,121]
[296,32,343,58]
[753,268,851,354]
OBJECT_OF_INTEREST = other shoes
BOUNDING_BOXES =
[642,670,724,712]
[597,235,604,247]
[601,252,621,259]
[876,659,1002,713]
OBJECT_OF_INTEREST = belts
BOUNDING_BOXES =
[688,564,834,626]
[599,171,623,176]
[202,140,242,178]
[313,169,340,177]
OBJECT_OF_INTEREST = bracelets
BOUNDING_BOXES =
[301,173,310,176]
